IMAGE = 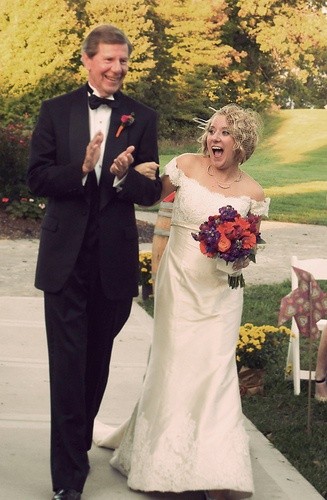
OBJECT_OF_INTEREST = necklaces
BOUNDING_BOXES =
[206,164,242,190]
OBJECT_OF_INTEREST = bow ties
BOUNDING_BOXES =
[84,81,118,112]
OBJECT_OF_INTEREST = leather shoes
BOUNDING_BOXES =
[52,488,80,500]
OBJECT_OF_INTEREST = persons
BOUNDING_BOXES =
[26,24,162,500]
[132,104,270,500]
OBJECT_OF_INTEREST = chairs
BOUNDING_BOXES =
[287,256,327,395]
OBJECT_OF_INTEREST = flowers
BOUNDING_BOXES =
[191,205,265,289]
[236,323,296,395]
[139,250,154,300]
[116,112,136,138]
